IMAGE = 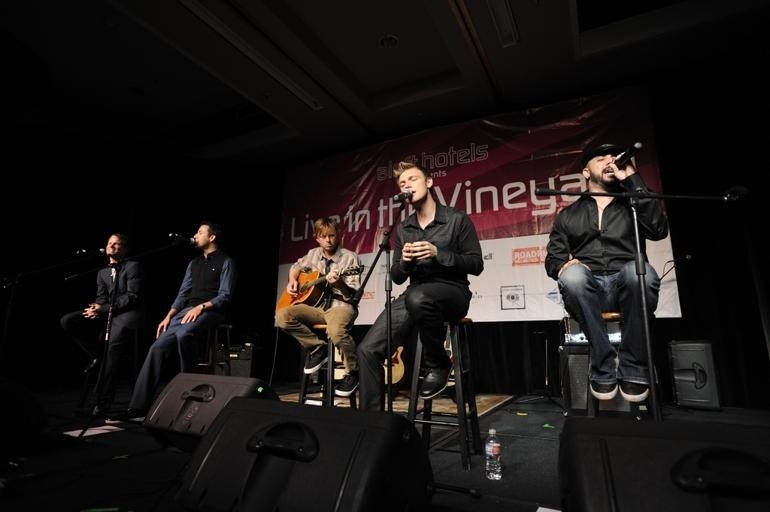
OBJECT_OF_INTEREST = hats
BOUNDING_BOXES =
[576,138,623,167]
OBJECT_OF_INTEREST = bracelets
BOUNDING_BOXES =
[199,302,206,310]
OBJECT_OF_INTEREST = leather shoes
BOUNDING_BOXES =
[84,358,99,373]
[420,360,454,400]
[104,408,145,422]
[92,401,111,418]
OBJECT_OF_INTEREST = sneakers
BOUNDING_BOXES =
[336,370,360,396]
[304,345,331,374]
[590,380,618,402]
[619,379,650,404]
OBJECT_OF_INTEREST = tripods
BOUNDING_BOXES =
[72,284,143,446]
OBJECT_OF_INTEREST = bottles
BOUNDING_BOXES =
[485,428,503,480]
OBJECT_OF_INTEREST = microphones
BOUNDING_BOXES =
[75,248,106,255]
[167,233,194,244]
[392,193,412,201]
[607,141,643,173]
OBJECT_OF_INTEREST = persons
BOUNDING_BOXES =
[354,160,482,412]
[545,134,667,403]
[104,224,237,424]
[274,217,363,397]
[60,233,150,419]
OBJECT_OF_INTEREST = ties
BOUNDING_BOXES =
[324,259,334,275]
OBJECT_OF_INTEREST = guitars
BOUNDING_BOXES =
[382,343,405,385]
[274,263,365,324]
[443,321,459,387]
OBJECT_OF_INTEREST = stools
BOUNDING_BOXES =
[408,319,482,470]
[586,312,661,418]
[192,325,233,376]
[298,324,356,407]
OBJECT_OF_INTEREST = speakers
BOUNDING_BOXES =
[666,340,722,412]
